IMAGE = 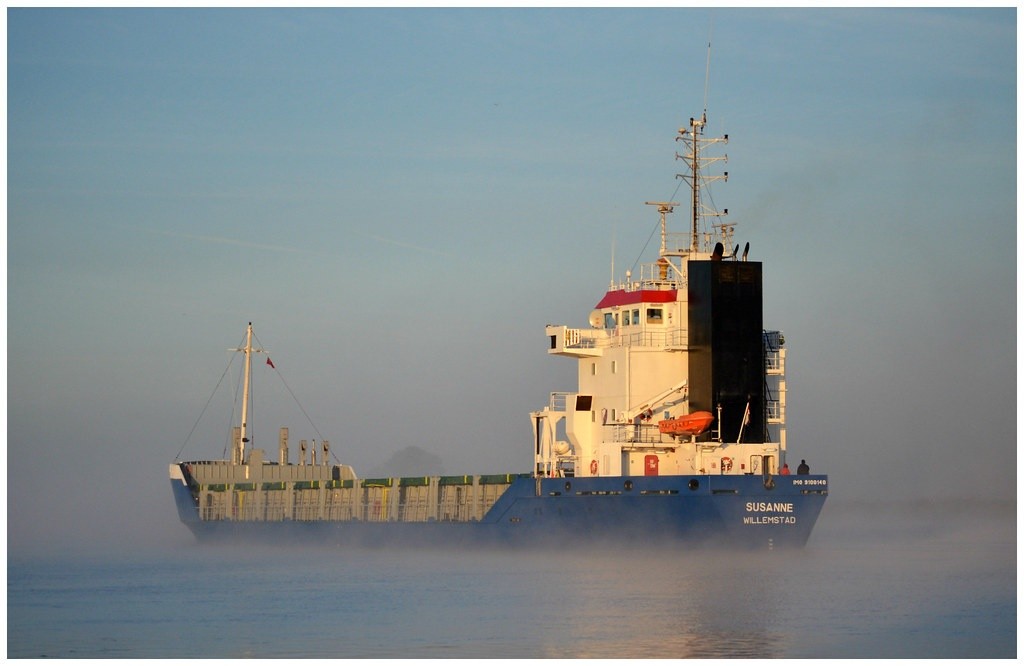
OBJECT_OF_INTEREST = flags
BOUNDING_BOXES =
[266,357,274,369]
[745,409,750,425]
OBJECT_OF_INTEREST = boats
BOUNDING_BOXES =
[169,43,829,551]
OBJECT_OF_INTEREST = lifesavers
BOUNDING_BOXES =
[637,406,654,423]
[589,459,600,475]
[720,456,733,471]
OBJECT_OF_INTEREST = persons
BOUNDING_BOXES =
[797,460,809,474]
[779,464,790,474]
